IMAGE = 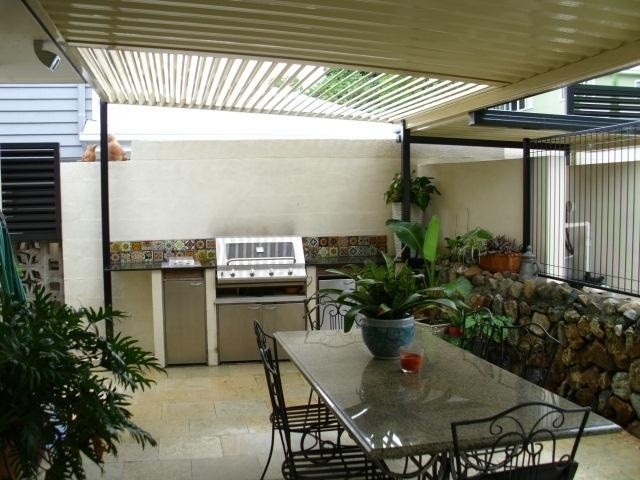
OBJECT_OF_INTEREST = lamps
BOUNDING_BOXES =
[32,39,60,72]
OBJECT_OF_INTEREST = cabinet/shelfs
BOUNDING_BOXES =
[218,302,307,364]
[163,269,206,366]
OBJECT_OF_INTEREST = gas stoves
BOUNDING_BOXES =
[216,238,306,285]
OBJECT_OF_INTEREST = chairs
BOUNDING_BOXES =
[260,348,395,480]
[450,400,591,480]
[254,319,348,478]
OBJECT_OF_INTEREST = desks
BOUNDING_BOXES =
[272,328,622,480]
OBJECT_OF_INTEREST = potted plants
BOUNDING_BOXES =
[316,214,524,360]
[0,275,169,480]
[383,170,443,259]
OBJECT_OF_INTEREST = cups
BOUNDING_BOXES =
[400,347,424,375]
